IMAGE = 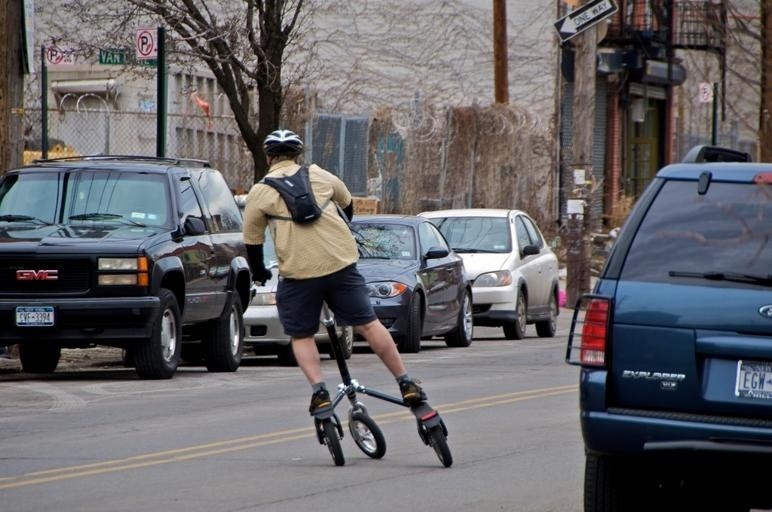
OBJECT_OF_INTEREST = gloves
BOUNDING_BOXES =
[252,267,272,286]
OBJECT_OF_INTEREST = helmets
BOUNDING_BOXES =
[263,128,303,153]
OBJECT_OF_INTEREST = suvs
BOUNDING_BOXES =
[564,144,770,510]
[1,153,256,381]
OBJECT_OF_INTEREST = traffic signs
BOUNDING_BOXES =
[99,48,157,66]
[553,0,619,42]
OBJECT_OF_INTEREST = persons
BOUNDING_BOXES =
[241,129,430,411]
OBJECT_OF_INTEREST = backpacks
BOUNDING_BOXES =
[256,163,331,226]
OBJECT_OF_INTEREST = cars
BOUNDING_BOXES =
[415,208,561,340]
[349,213,474,353]
[178,195,354,366]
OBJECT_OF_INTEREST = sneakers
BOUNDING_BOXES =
[309,388,332,415]
[399,377,428,402]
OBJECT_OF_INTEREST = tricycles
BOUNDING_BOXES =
[252,233,453,471]
